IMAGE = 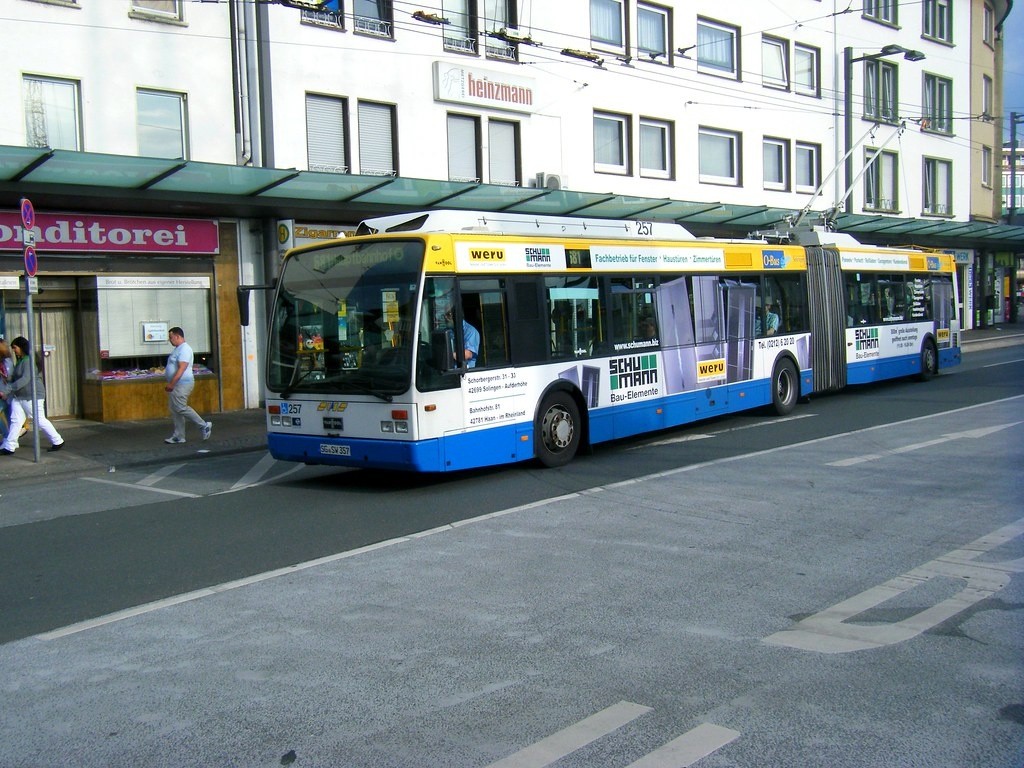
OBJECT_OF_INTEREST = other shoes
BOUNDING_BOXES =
[0,448,15,456]
[47,441,65,451]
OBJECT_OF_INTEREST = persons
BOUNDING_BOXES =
[641,314,659,343]
[444,301,479,369]
[756,301,779,336]
[165,327,213,443]
[0,337,65,455]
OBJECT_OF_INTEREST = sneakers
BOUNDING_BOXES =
[165,435,187,443]
[201,422,212,440]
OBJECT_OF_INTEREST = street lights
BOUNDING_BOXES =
[843,44,926,217]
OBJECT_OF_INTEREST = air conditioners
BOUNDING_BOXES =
[535,171,561,190]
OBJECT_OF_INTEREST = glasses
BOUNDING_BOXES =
[444,312,452,317]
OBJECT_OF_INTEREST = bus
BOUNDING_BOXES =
[266,118,963,480]
